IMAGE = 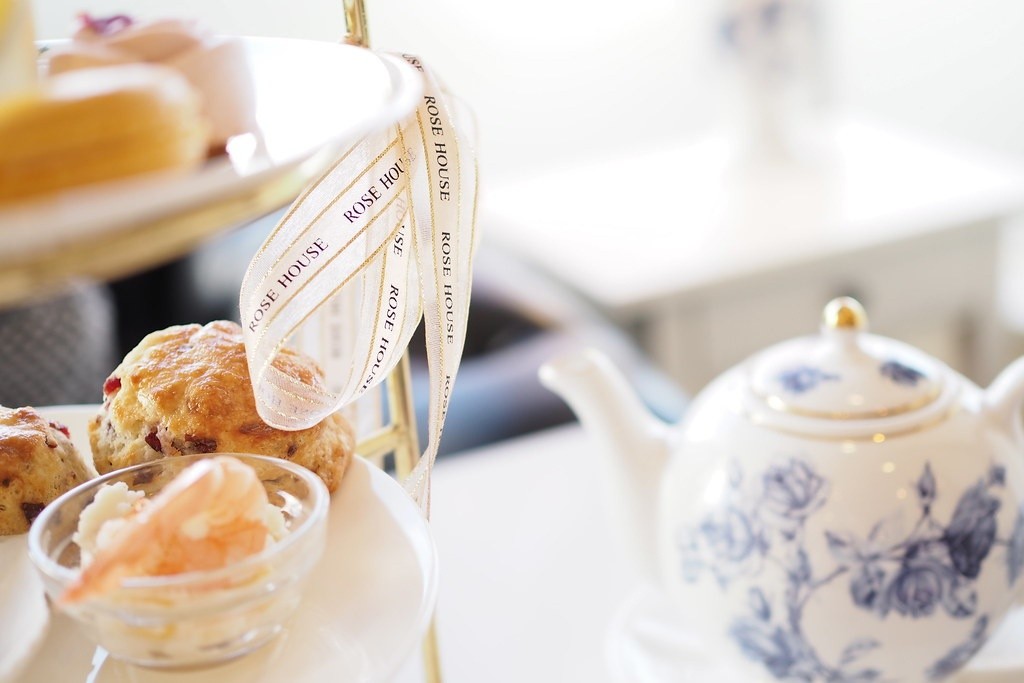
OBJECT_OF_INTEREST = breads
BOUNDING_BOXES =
[0,65,210,208]
[86,320,354,501]
[0,405,94,538]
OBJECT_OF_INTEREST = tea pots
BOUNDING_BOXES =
[533,295,1024,683]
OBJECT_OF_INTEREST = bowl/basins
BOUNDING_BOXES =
[28,453,331,671]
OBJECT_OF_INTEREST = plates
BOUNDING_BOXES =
[0,35,429,307]
[0,401,437,683]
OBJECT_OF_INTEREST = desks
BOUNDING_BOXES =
[476,114,1021,398]
